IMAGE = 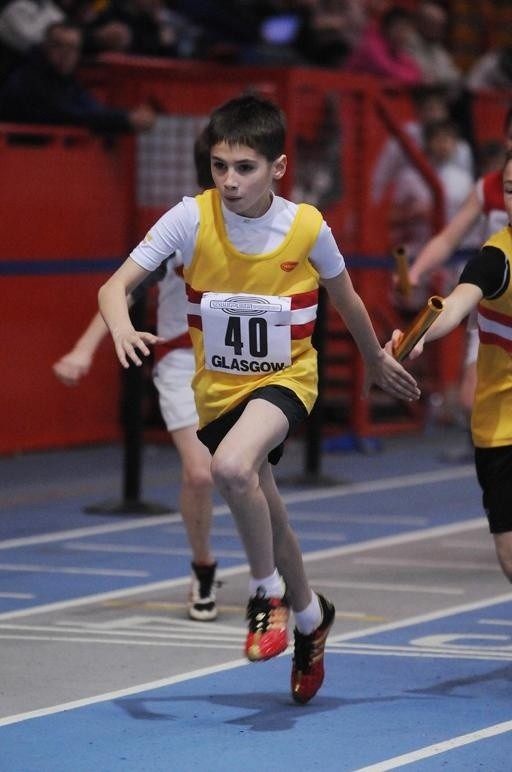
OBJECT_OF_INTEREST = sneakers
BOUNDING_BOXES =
[290,592,337,705]
[189,559,222,623]
[242,572,291,664]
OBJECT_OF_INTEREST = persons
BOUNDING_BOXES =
[98,89,420,706]
[390,104,511,448]
[0,0,512,304]
[54,127,289,626]
[383,154,510,584]
